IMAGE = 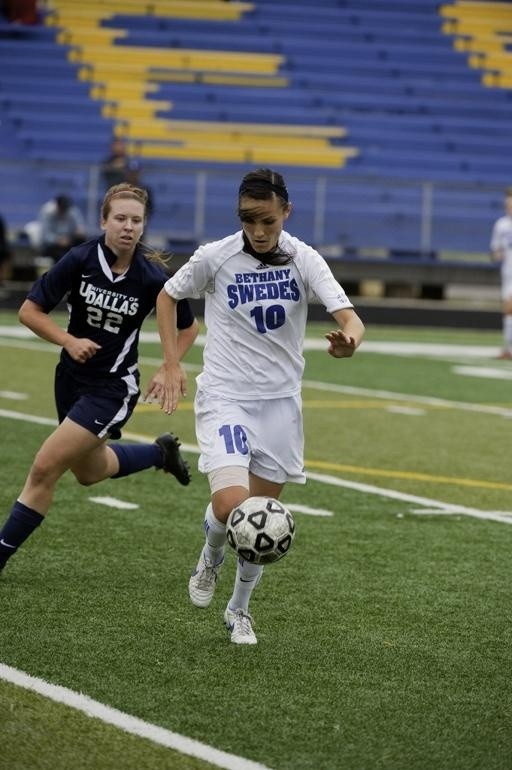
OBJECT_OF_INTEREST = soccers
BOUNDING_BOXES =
[226,497,297,564]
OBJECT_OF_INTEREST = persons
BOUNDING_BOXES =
[152,168,366,646]
[34,192,86,267]
[98,139,132,190]
[489,186,511,360]
[0,182,199,576]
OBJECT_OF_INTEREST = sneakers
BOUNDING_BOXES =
[154,431,192,485]
[188,545,225,609]
[222,607,258,645]
